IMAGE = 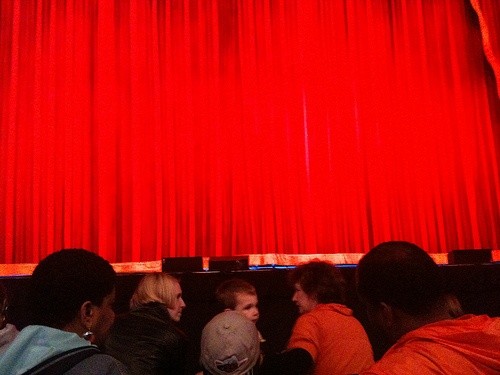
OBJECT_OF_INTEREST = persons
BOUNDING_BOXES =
[0,240,500,375]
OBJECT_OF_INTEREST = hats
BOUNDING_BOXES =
[199,311,260,375]
